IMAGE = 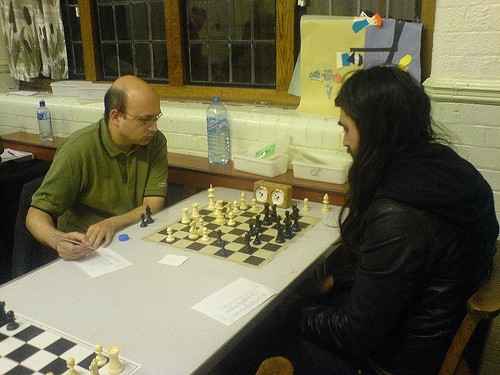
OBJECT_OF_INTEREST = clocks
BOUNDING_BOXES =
[254,181,292,208]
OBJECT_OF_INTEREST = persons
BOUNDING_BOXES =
[25,75,169,267]
[237,66,500,375]
[187,0,275,84]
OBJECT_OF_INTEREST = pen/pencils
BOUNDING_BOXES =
[6,150,16,157]
[51,234,96,251]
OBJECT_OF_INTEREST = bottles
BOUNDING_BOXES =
[37,101,54,142]
[207,97,230,164]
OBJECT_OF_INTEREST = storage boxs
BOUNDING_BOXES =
[231,134,353,185]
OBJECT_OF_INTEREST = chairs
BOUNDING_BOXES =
[11,174,50,282]
[256,240,500,375]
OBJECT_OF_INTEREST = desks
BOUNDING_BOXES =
[0,186,354,375]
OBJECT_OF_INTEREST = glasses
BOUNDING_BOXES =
[121,110,163,127]
